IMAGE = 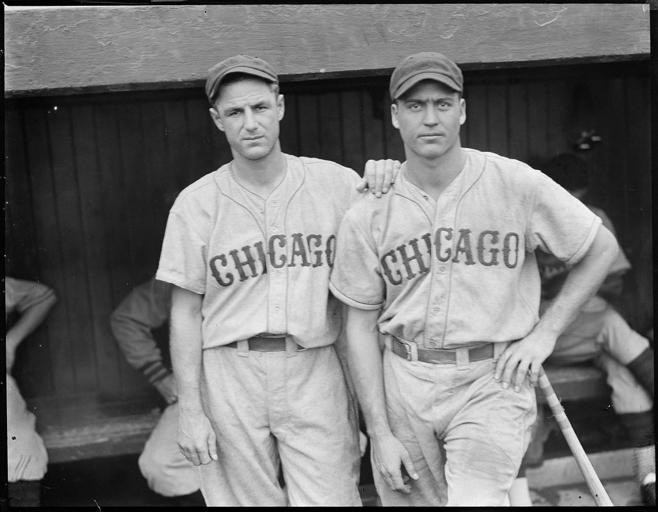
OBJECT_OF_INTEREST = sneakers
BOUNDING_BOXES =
[639,481,656,506]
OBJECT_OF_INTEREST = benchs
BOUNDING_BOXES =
[29,354,640,489]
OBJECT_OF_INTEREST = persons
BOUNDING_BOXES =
[533,156,655,507]
[108,268,369,510]
[327,52,619,508]
[4,276,59,508]
[152,52,405,506]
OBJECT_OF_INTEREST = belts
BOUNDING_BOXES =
[221,331,306,355]
[391,333,495,366]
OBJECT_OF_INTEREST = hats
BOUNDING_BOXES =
[205,53,279,106]
[388,50,464,102]
